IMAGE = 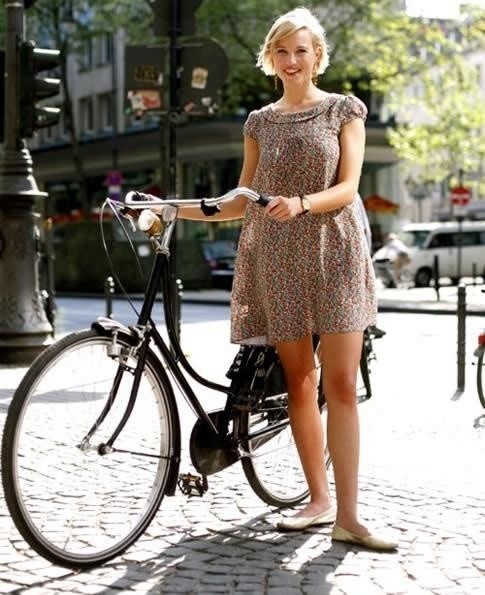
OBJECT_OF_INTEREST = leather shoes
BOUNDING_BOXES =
[330,523,399,551]
[276,507,338,531]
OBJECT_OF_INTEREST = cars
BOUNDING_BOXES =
[200,239,237,287]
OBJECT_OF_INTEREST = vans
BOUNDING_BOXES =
[373,221,485,289]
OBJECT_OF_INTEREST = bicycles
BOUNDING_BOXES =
[472,329,485,409]
[360,325,386,397]
[0,187,333,570]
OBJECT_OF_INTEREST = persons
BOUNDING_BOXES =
[117,3,402,552]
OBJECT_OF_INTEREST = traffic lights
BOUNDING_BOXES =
[19,40,61,139]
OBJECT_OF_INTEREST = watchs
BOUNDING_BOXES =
[295,193,310,215]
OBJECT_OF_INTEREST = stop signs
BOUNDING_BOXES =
[450,187,470,206]
